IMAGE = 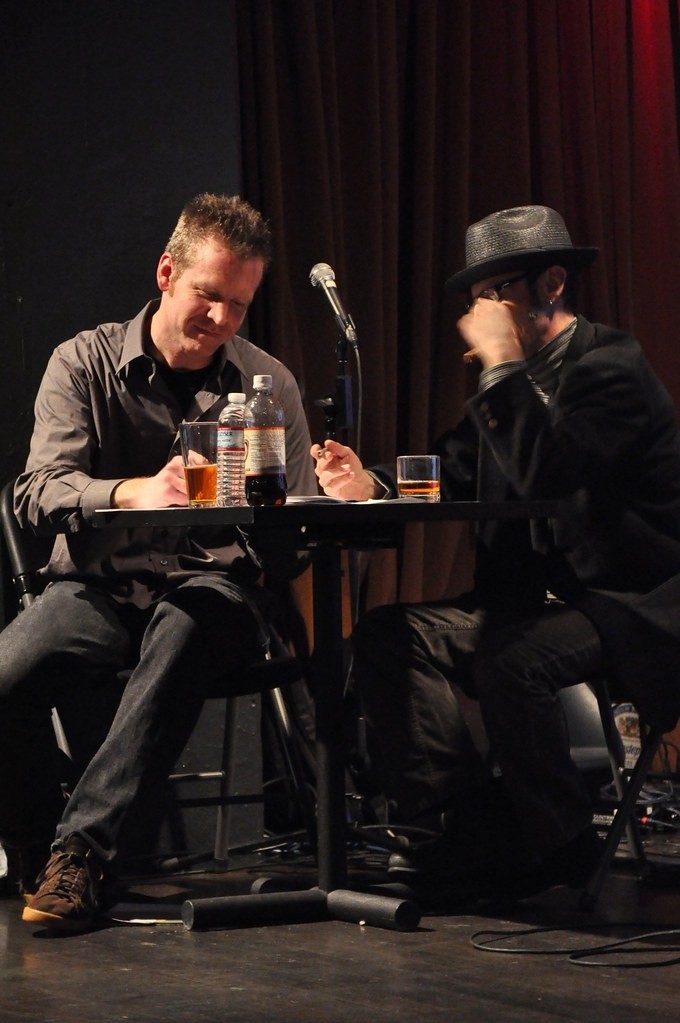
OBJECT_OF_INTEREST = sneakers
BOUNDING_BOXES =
[19,844,121,931]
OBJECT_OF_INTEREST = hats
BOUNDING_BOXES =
[440,203,601,295]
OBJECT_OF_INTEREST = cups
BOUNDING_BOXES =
[178,418,217,507]
[397,454,441,502]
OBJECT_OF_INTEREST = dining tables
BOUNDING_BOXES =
[88,495,491,934]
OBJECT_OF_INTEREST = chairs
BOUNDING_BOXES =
[0,472,318,905]
[561,653,674,917]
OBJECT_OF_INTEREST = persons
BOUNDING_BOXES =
[0,192,319,926]
[306,203,673,881]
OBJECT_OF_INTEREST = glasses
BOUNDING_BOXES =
[462,265,550,310]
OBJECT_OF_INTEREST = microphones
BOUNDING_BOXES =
[309,262,359,348]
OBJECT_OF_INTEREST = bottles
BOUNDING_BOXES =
[218,391,256,508]
[244,374,289,507]
